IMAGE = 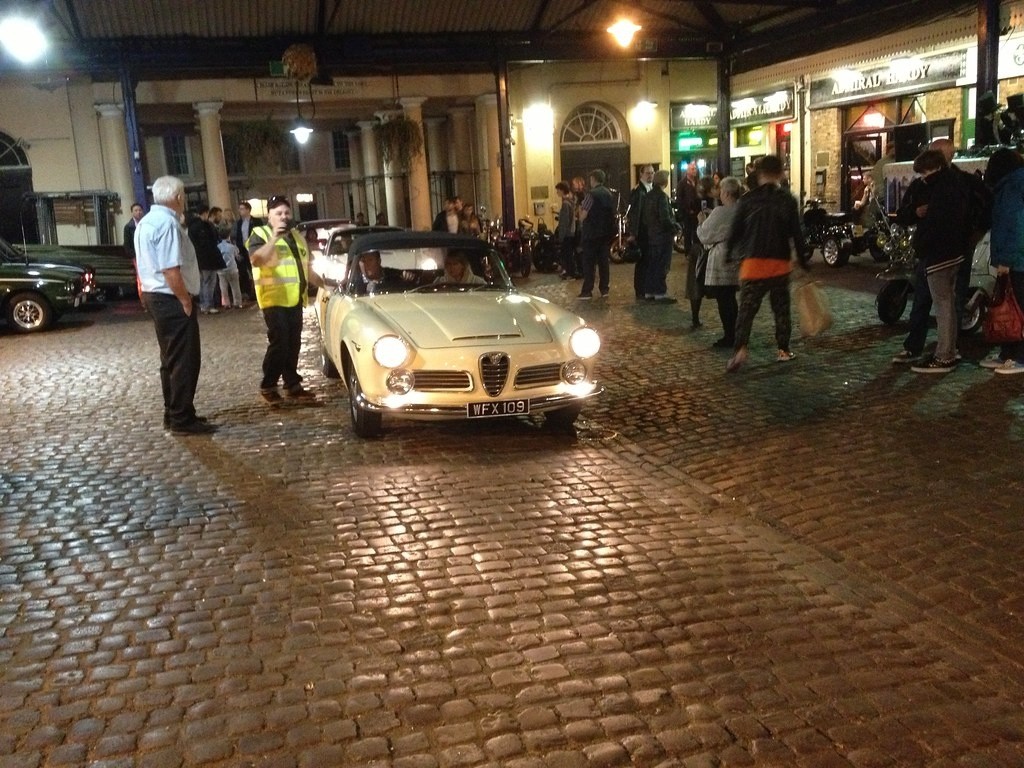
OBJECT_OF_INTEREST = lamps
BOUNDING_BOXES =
[290,81,313,146]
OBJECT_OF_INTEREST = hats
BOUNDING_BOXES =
[267,194,288,209]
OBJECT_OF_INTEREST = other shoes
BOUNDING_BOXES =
[712,336,735,347]
[892,349,922,363]
[260,387,284,404]
[644,291,678,301]
[160,405,219,435]
[576,292,593,300]
[285,387,315,399]
[601,290,609,297]
[200,307,220,314]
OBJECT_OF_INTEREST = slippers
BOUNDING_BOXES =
[726,348,750,374]
[777,351,796,361]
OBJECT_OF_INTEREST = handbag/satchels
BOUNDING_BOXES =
[981,269,1023,342]
[694,249,708,280]
[794,270,834,339]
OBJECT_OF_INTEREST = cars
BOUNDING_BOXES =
[314,230,606,439]
[296,219,406,289]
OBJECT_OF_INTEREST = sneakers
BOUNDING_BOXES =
[909,353,956,373]
[979,355,1024,374]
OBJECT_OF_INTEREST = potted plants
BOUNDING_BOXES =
[372,114,422,179]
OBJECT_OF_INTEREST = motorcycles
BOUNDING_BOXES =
[609,187,689,264]
[872,226,1000,334]
[485,220,563,277]
[798,197,893,267]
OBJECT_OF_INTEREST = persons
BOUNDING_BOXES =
[857,136,1024,375]
[351,247,421,295]
[553,155,812,373]
[432,251,488,285]
[355,196,483,236]
[304,227,319,242]
[124,175,325,437]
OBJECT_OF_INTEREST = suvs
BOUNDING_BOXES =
[0,239,99,334]
